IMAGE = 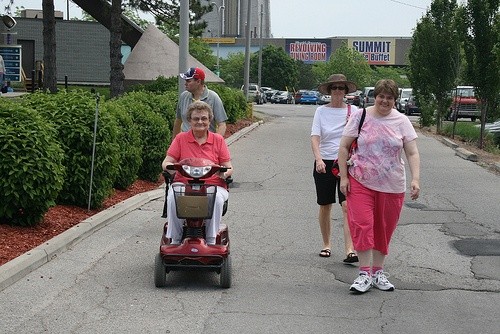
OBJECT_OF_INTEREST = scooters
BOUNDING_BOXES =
[154,158,233,288]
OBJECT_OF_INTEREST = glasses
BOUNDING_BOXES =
[330,86,346,90]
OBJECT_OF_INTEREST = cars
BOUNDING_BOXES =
[271,91,292,104]
[405,96,422,116]
[359,87,375,108]
[395,88,413,113]
[447,86,487,122]
[320,90,363,105]
[261,87,279,103]
[242,83,263,104]
[295,90,306,104]
[300,91,321,105]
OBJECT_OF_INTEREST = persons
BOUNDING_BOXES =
[337,80,421,293]
[162,101,233,246]
[310,74,360,263]
[173,67,228,140]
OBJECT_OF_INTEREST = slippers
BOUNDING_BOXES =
[319,249,331,257]
[343,253,359,263]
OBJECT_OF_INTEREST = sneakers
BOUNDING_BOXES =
[371,269,395,291]
[349,270,374,293]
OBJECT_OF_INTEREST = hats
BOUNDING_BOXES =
[318,73,357,94]
[180,67,205,81]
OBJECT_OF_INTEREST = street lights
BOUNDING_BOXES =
[211,2,226,72]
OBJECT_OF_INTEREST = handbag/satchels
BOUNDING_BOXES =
[331,107,366,178]
